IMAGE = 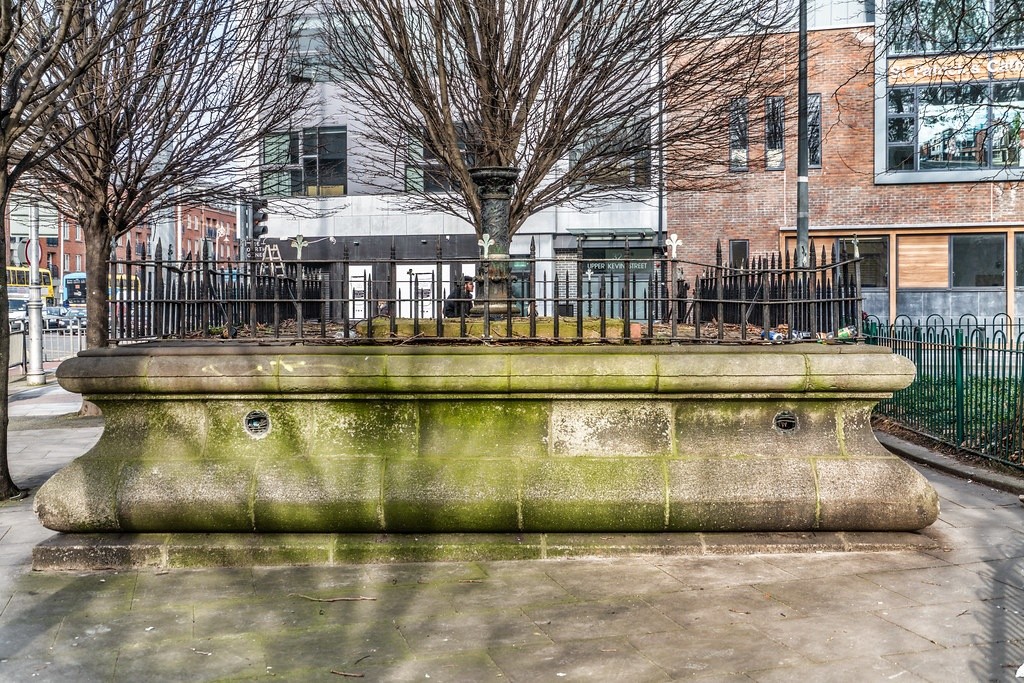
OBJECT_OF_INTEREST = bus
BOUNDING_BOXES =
[6,266,55,306]
[62,272,141,320]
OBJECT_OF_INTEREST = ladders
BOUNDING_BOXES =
[259,244,289,284]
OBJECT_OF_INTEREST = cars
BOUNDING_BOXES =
[8,299,88,336]
[124,306,147,337]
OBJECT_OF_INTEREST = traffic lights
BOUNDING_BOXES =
[252,198,268,239]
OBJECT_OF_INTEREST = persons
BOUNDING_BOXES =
[441,276,475,317]
